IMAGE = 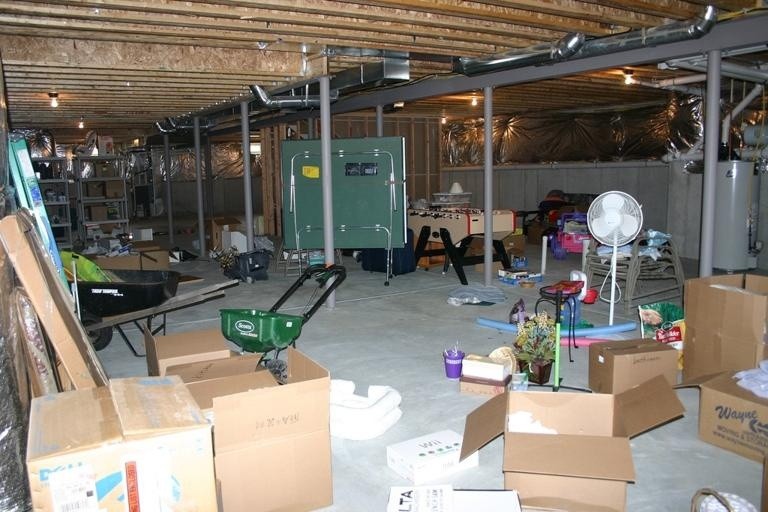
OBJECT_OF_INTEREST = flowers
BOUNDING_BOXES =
[511,308,557,370]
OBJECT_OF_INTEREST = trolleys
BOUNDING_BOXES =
[219,259,349,363]
[55,250,179,354]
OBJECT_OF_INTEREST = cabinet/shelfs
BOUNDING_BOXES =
[77,155,130,242]
[31,156,75,252]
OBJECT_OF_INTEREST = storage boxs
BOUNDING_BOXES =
[587,335,682,396]
[86,180,104,197]
[105,180,124,200]
[454,368,688,511]
[1,207,108,388]
[94,161,116,178]
[100,224,118,233]
[207,215,242,250]
[683,269,767,381]
[25,323,331,511]
[80,228,171,273]
[672,370,768,462]
[89,204,108,221]
[466,225,547,272]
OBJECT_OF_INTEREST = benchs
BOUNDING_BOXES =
[515,188,598,245]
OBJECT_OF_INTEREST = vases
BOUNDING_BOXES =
[517,358,555,387]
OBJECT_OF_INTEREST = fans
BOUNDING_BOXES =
[585,188,645,327]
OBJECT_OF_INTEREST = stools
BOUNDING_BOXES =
[534,285,581,364]
[585,230,687,314]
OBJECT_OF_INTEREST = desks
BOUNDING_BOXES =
[278,134,408,290]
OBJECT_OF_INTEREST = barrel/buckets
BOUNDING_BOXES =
[443,350,466,378]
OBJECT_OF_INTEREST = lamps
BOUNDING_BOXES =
[622,67,635,87]
[48,91,64,108]
